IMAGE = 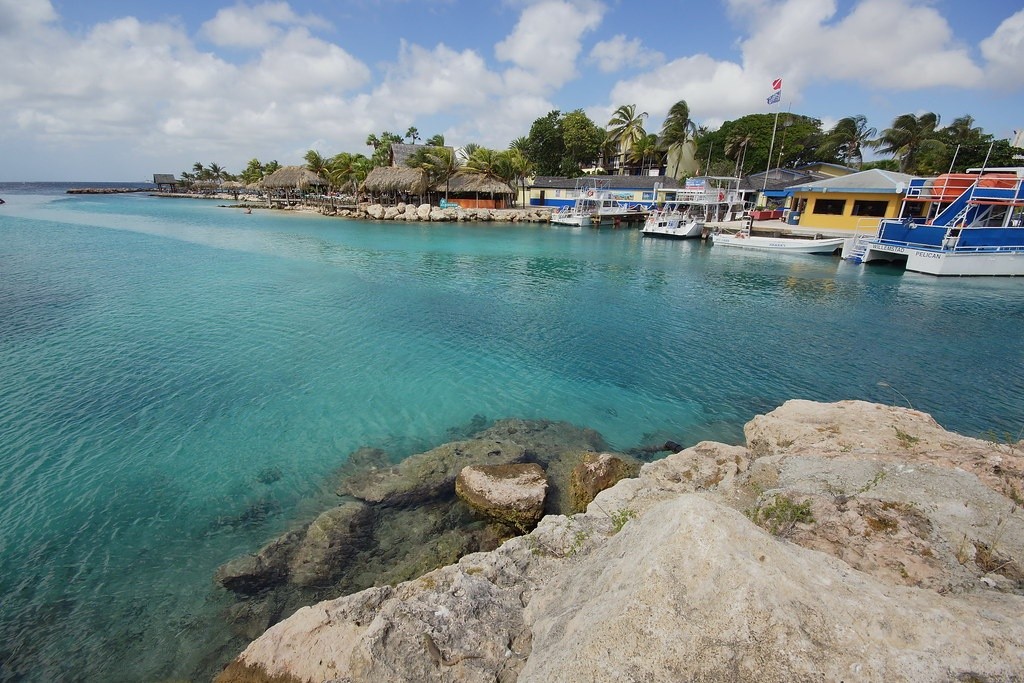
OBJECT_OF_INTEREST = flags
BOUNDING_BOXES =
[767,90,781,106]
[772,79,781,89]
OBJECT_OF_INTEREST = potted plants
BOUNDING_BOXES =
[749,203,784,221]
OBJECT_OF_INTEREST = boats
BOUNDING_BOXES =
[840,142,1024,277]
[550,177,625,227]
[638,200,708,238]
[708,232,847,254]
